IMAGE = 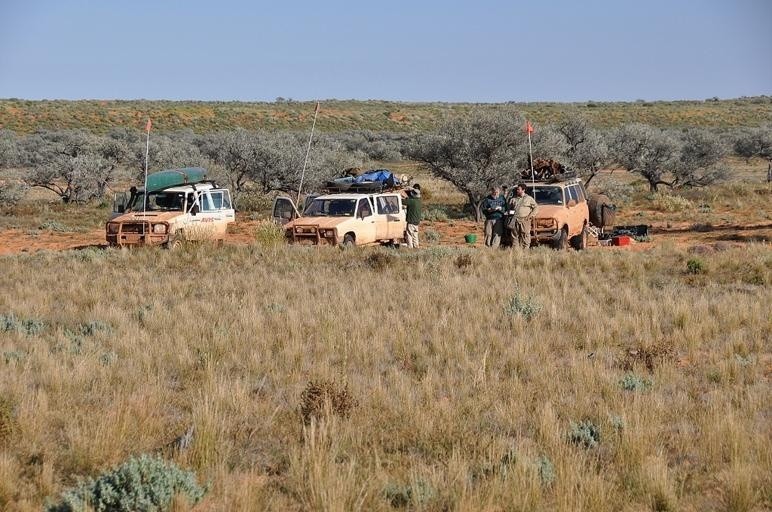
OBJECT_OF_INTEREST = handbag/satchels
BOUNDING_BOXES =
[504,214,516,230]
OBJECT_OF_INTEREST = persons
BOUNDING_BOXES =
[482,185,507,249]
[509,183,538,249]
[402,189,421,248]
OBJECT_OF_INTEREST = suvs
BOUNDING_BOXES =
[272,190,407,250]
[106,181,235,251]
[504,176,590,250]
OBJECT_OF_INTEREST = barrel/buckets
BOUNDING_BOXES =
[464,233,477,244]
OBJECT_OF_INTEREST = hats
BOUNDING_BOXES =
[410,189,421,198]
